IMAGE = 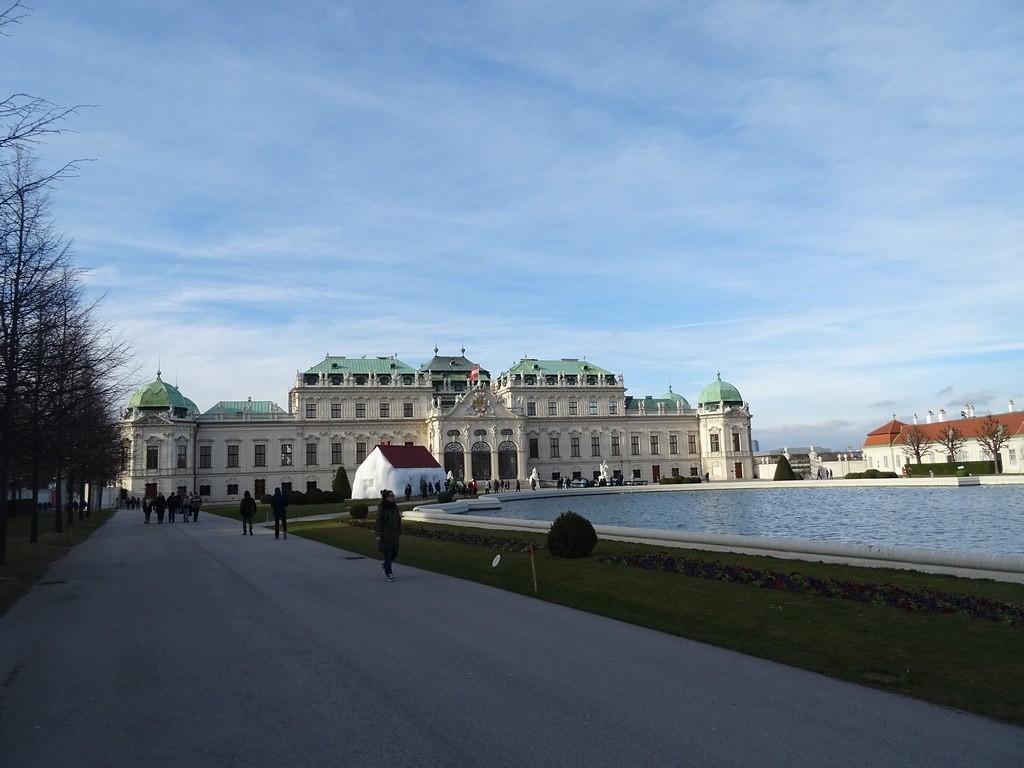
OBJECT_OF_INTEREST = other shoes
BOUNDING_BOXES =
[283,531,287,539]
[276,535,278,539]
[250,531,253,535]
[381,563,395,572]
[386,577,396,582]
[242,533,246,535]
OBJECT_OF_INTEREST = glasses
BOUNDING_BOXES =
[387,495,395,497]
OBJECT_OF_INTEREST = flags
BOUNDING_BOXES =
[470,367,479,379]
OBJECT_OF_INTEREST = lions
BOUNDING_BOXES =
[529,467,540,480]
[446,470,454,480]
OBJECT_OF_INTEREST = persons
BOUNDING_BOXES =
[421,478,477,500]
[270,487,287,539]
[405,484,412,501]
[125,491,202,524]
[374,489,401,582]
[585,473,624,488]
[73,500,88,511]
[39,501,52,512]
[516,480,520,492]
[817,470,822,479]
[557,476,571,489]
[485,479,510,494]
[531,479,536,491]
[240,491,257,536]
[826,469,833,479]
[908,467,912,477]
